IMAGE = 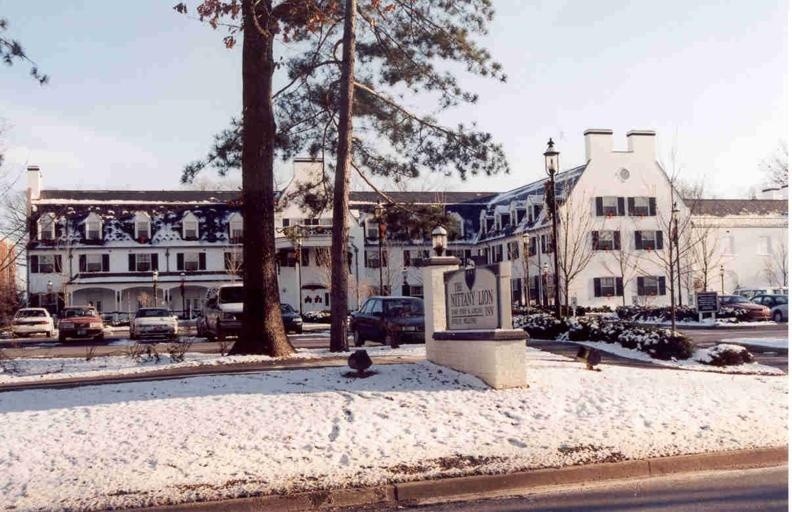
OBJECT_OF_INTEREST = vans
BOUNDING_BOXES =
[204,284,244,342]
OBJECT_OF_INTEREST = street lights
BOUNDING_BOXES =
[431,220,447,257]
[178,270,187,319]
[542,262,550,305]
[46,280,53,317]
[152,269,158,307]
[521,228,531,308]
[277,224,311,316]
[544,136,562,321]
[671,201,684,306]
[720,265,725,294]
[374,198,386,294]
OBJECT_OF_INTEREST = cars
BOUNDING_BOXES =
[348,295,425,348]
[715,286,788,322]
[194,310,204,336]
[56,305,106,343]
[279,303,304,334]
[12,307,55,338]
[129,306,180,340]
